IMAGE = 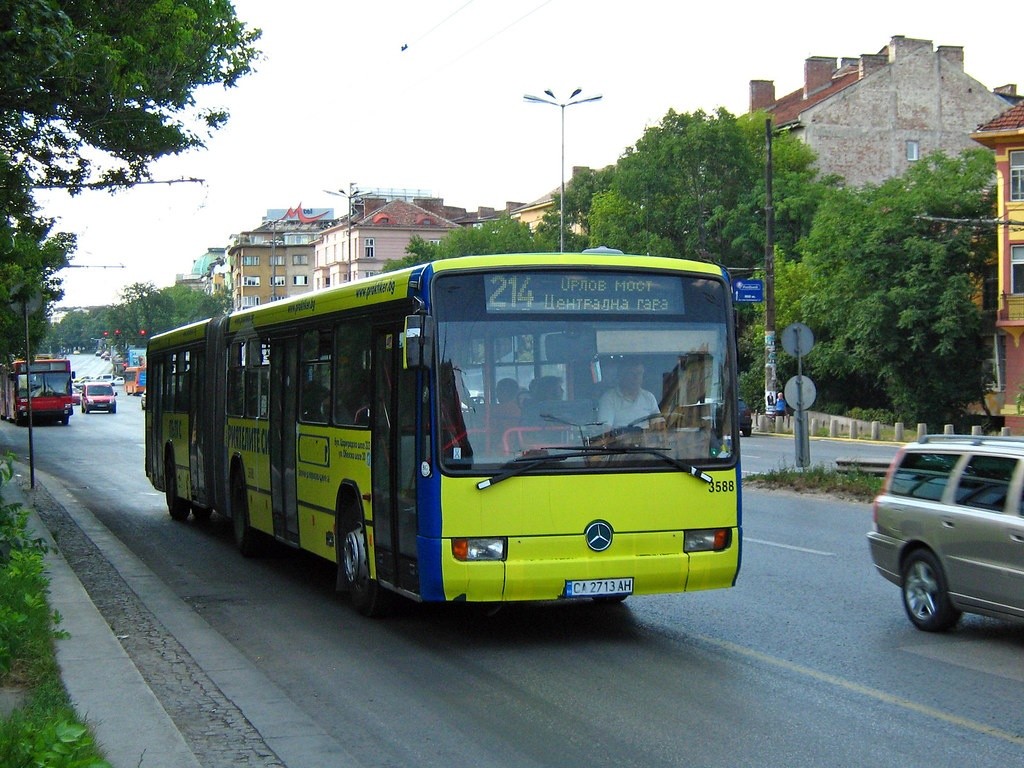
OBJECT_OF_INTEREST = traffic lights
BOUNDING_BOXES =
[140,329,147,336]
[115,328,122,335]
[103,329,109,337]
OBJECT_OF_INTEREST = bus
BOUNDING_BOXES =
[145,245,745,621]
[123,366,147,397]
[0,353,75,426]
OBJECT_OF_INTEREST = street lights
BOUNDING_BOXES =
[321,181,374,283]
[522,87,604,252]
[763,115,805,414]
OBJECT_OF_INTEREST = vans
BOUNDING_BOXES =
[81,382,118,414]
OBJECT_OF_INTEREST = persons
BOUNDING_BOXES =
[597,357,666,439]
[520,376,564,427]
[490,379,522,454]
[775,392,786,421]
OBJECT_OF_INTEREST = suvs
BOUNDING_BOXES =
[738,397,753,438]
[867,434,1024,635]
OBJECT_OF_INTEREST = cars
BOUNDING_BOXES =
[73,374,126,390]
[71,386,81,405]
[141,388,147,410]
[74,350,80,355]
[96,349,111,361]
